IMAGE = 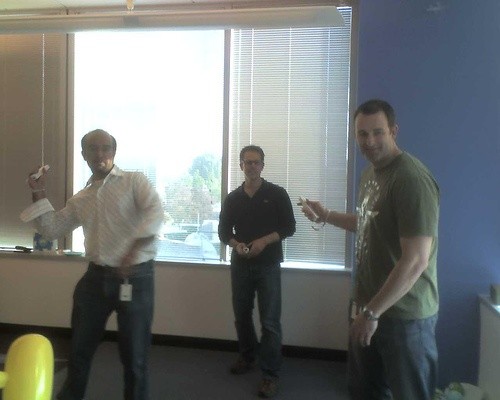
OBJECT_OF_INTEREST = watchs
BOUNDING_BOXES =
[362,306,379,321]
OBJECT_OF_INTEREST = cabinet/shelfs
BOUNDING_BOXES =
[476,293,500,400]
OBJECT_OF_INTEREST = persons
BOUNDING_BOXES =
[20,128,165,400]
[297,98,441,399]
[218,145,296,399]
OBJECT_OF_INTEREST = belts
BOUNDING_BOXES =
[89,260,155,275]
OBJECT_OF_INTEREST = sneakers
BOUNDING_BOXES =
[230,354,255,374]
[259,380,280,397]
